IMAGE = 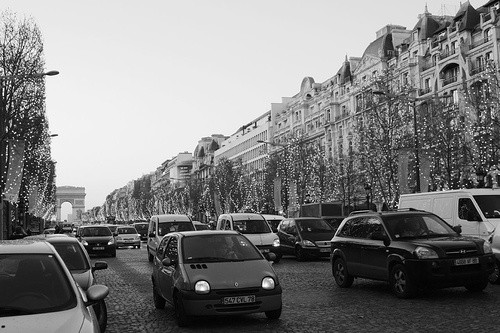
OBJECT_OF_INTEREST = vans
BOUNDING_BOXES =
[147,214,197,262]
[216,213,281,263]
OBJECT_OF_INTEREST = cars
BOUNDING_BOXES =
[114,226,141,249]
[16,234,109,333]
[277,217,336,261]
[0,239,109,333]
[152,230,283,327]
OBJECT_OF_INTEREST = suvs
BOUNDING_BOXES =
[330,207,494,299]
[75,225,118,257]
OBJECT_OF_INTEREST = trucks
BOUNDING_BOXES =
[397,188,500,283]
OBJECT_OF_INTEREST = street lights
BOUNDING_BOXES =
[372,89,420,192]
[257,140,287,210]
[0,70,59,239]
